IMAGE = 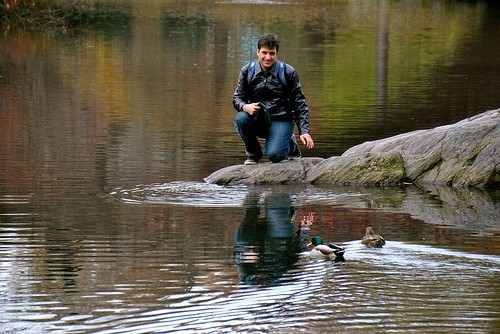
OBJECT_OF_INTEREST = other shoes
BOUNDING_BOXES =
[288,134,301,159]
[244,158,259,165]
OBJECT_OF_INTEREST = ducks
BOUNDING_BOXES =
[361,226,386,245]
[305,236,347,258]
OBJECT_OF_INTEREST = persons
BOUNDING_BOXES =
[233,191,315,288]
[231,33,316,164]
